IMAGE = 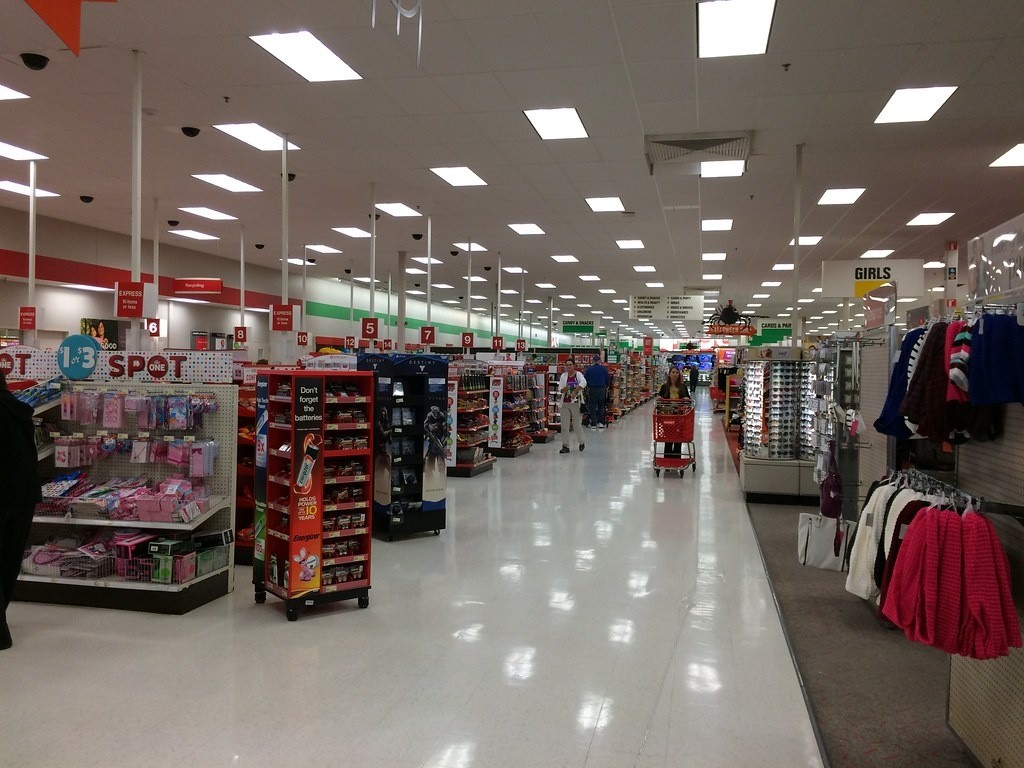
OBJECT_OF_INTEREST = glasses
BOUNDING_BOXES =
[738,360,836,461]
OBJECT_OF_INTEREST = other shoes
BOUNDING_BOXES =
[579,443,584,451]
[593,355,600,360]
[560,446,569,453]
[599,422,605,427]
[592,423,598,428]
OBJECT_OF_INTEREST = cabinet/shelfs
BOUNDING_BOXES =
[234,384,256,566]
[355,350,661,541]
[724,374,742,433]
[1,376,239,616]
[255,370,375,624]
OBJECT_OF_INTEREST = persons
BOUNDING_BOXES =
[0,372,41,650]
[690,366,699,392]
[558,359,587,453]
[659,366,691,472]
[584,355,610,429]
[89,320,109,351]
[506,354,512,361]
[220,340,225,349]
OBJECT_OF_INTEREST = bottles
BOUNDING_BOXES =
[459,373,486,390]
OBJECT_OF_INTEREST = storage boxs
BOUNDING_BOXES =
[114,527,234,584]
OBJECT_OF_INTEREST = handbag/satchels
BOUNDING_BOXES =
[797,512,857,572]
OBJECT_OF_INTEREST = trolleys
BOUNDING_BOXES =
[653,397,696,477]
